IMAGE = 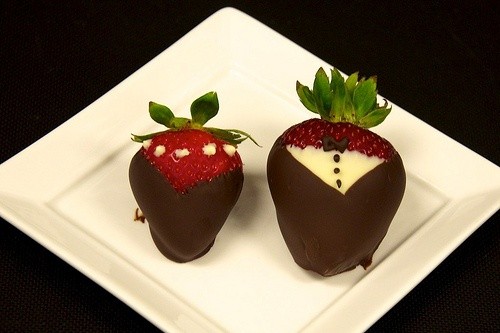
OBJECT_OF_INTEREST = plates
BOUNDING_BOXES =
[0,7,500,333]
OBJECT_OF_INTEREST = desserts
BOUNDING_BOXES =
[127,90,246,263]
[265,66,408,277]
